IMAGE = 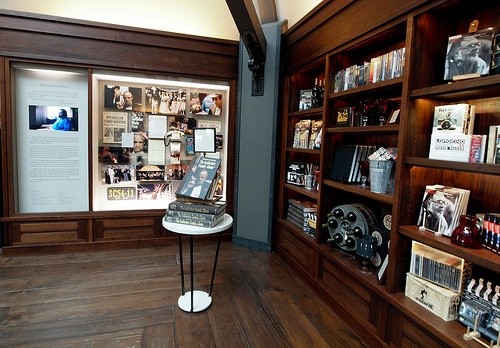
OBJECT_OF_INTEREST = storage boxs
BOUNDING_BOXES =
[405,271,464,321]
[408,241,471,294]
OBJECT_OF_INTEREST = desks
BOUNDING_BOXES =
[162,212,233,314]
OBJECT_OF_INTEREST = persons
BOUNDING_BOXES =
[48,109,78,131]
[99,86,223,201]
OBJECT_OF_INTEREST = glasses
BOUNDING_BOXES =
[432,199,448,207]
[461,42,481,50]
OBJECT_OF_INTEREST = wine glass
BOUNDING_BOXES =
[359,99,373,127]
[375,99,391,126]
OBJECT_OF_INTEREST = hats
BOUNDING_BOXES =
[211,93,222,99]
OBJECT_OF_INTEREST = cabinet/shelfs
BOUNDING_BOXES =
[274,1,500,348]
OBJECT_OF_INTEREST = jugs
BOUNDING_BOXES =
[450,215,484,249]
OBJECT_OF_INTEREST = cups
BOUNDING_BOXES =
[304,175,314,189]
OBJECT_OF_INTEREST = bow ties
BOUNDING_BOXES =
[196,183,203,186]
[126,104,132,107]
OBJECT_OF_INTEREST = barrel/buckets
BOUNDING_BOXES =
[368,159,394,193]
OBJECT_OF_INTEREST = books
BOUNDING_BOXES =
[293,120,322,149]
[444,27,495,80]
[299,90,312,111]
[429,104,476,162]
[337,106,351,125]
[417,186,471,237]
[482,214,500,256]
[287,199,317,231]
[334,47,405,93]
[329,145,376,182]
[470,126,500,164]
[165,196,228,228]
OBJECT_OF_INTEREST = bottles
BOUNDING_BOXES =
[341,210,369,222]
[327,209,344,218]
[327,233,343,244]
[346,225,373,237]
[321,219,338,229]
[339,237,355,249]
[312,77,325,109]
